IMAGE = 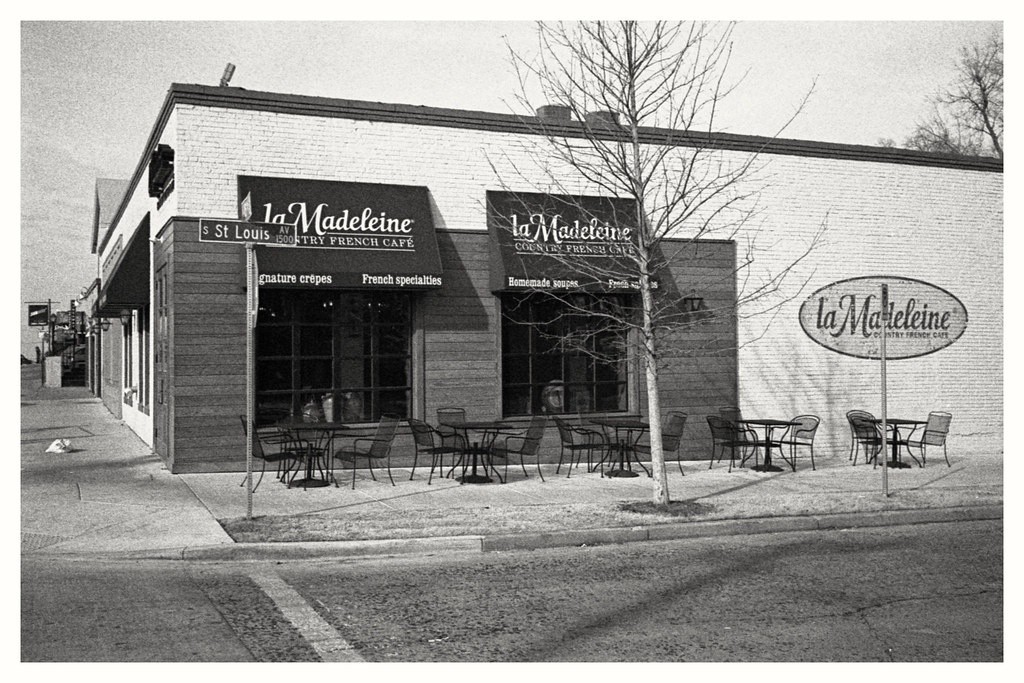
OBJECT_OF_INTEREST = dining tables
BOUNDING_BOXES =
[281,421,351,489]
[589,419,650,478]
[440,420,513,485]
[872,418,928,468]
[735,419,803,474]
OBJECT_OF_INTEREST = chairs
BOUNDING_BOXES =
[437,407,489,478]
[277,411,326,484]
[771,414,821,471]
[901,411,952,468]
[491,415,550,482]
[718,406,765,469]
[707,414,757,474]
[239,414,310,492]
[553,415,605,478]
[846,409,903,467]
[408,418,467,486]
[335,412,401,490]
[632,410,688,475]
